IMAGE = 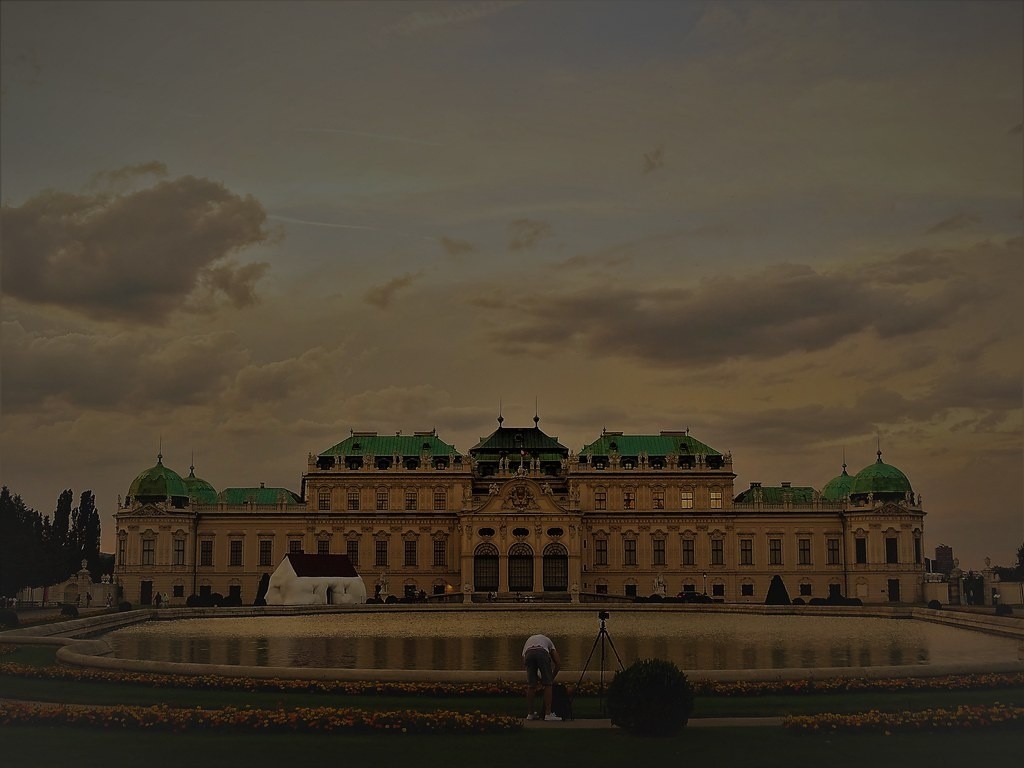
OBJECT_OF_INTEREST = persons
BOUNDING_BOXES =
[492,590,497,603]
[522,634,562,721]
[533,594,535,602]
[529,594,533,602]
[163,593,169,608]
[107,593,113,607]
[488,590,492,603]
[154,592,163,609]
[525,594,528,602]
[86,592,91,608]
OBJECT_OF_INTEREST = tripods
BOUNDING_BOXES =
[569,620,627,719]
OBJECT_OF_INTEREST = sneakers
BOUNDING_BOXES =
[545,713,562,721]
[527,712,540,720]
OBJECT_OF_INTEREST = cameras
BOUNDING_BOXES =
[598,609,610,620]
